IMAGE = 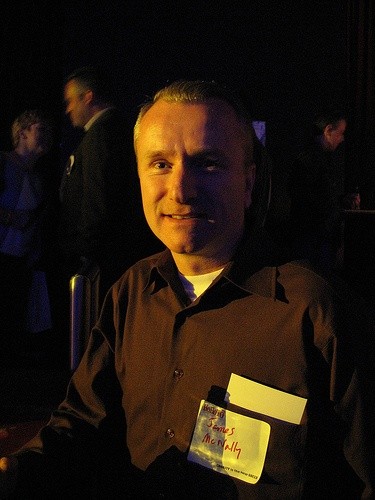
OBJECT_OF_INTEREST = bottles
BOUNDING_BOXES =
[351,183,362,208]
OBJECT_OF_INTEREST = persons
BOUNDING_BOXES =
[0,81,375,500]
[59,65,140,298]
[0,108,66,334]
[292,113,361,263]
